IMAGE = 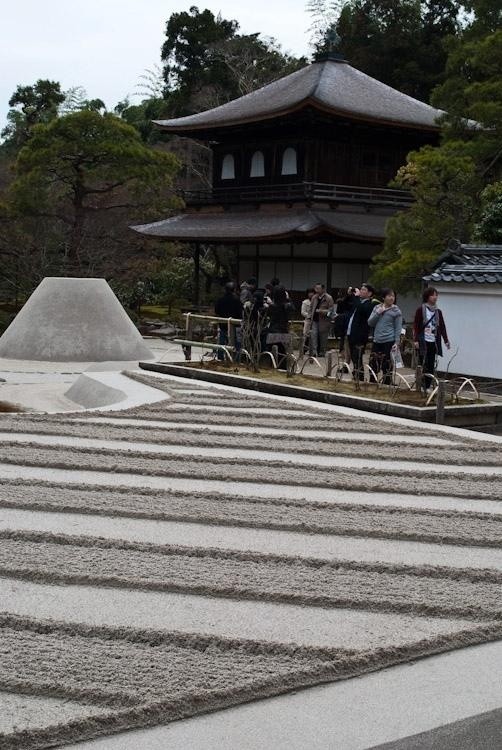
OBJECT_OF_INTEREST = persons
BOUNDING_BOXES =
[412,287,450,392]
[367,286,402,386]
[215,277,353,369]
[345,283,374,382]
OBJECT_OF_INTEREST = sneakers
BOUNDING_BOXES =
[337,367,398,386]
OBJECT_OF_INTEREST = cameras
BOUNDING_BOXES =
[351,289,356,293]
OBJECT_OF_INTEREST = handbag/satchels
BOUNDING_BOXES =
[321,310,336,320]
[391,347,405,369]
